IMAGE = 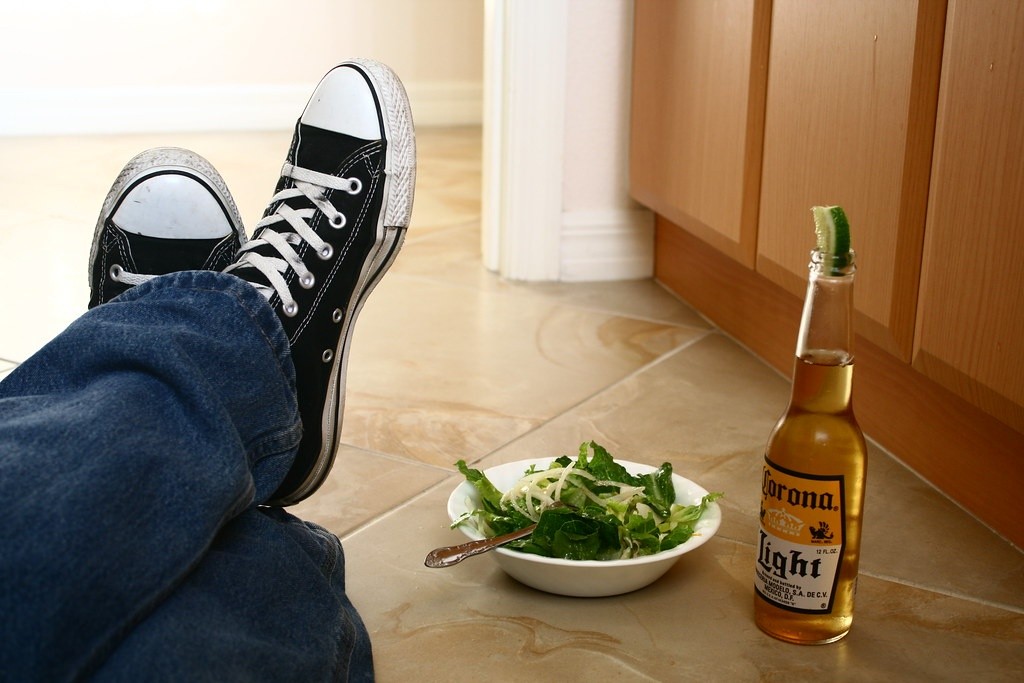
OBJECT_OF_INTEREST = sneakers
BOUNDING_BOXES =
[89,146,247,319]
[229,57,414,504]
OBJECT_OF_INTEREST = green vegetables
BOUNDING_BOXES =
[451,442,725,561]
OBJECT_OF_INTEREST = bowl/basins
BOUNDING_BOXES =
[447,456,722,597]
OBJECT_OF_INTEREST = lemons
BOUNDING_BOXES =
[813,206,851,277]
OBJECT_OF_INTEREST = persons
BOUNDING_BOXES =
[1,57,415,683]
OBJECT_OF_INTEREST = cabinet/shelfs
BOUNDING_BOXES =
[626,0,1024,552]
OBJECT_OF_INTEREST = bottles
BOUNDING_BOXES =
[754,247,868,644]
